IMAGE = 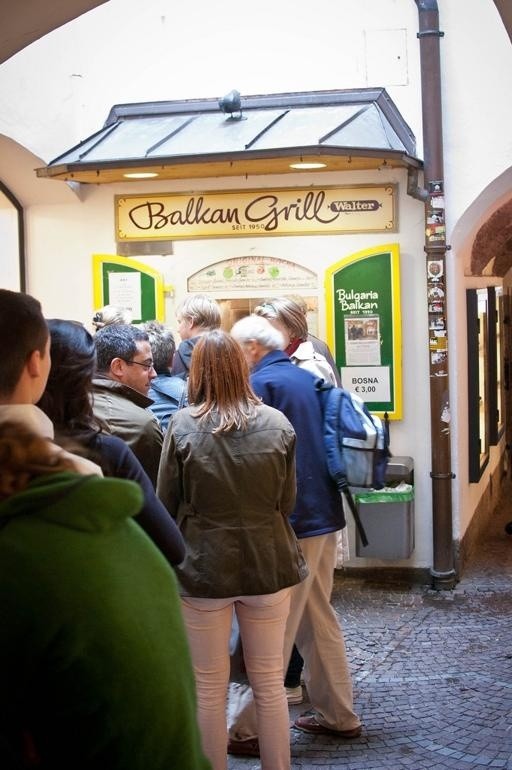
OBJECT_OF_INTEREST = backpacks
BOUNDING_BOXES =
[313,375,393,546]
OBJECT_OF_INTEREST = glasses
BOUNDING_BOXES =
[284,685,304,704]
[124,360,156,371]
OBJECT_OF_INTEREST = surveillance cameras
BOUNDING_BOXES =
[218,89,242,122]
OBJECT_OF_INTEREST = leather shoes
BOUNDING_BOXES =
[227,744,263,755]
[295,716,361,738]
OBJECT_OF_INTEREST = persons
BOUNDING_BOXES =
[89,324,159,494]
[37,317,187,567]
[156,331,309,768]
[1,287,105,477]
[255,296,337,388]
[228,312,362,755]
[0,418,214,770]
[172,295,221,380]
[141,319,189,431]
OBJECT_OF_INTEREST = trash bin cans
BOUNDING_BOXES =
[353,456,416,561]
